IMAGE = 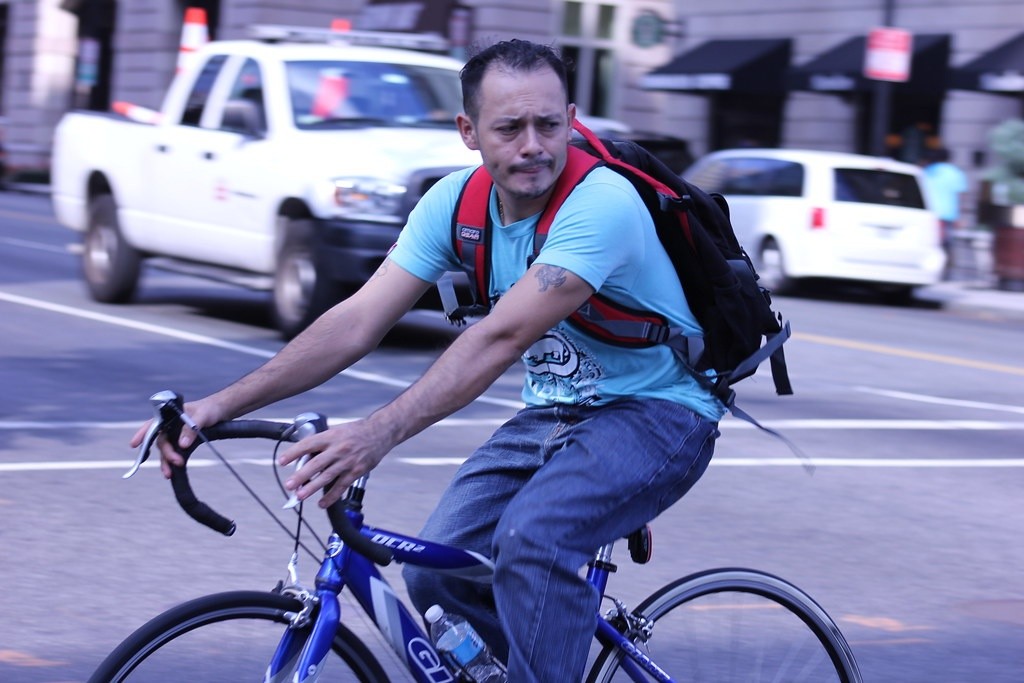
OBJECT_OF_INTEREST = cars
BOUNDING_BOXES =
[674,148,946,302]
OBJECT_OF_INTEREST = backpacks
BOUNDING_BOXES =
[452,116,816,474]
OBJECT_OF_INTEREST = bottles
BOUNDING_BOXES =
[425,603,507,683]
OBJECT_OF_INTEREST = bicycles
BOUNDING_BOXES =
[86,388,863,683]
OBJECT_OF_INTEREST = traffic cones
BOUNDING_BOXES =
[110,99,164,125]
[177,5,211,89]
[310,20,349,115]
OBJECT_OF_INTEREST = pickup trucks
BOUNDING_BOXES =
[46,23,490,341]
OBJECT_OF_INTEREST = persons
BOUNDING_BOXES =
[128,34,729,683]
[886,118,976,284]
